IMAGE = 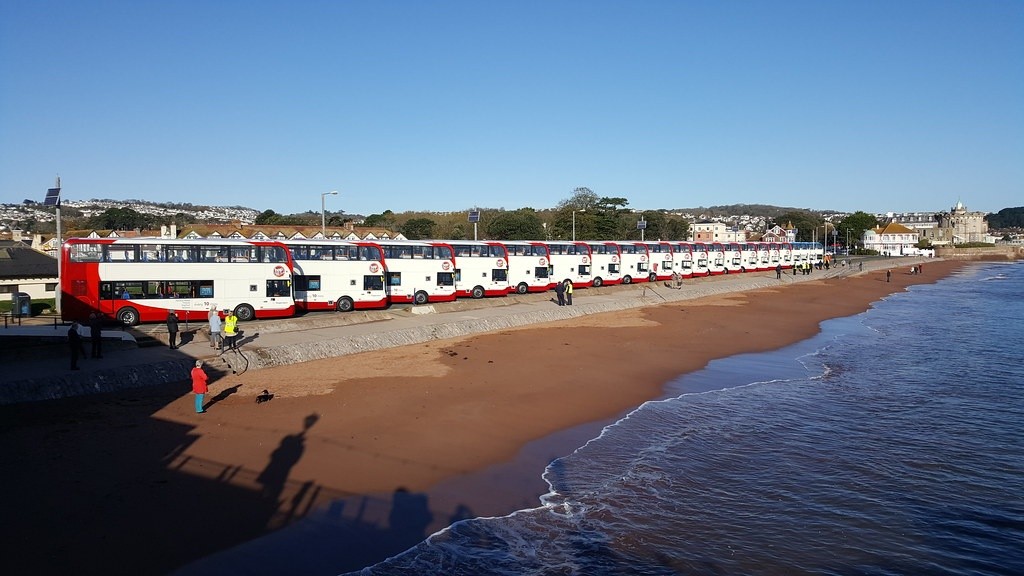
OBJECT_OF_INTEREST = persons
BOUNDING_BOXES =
[834,258,837,268]
[208,310,222,350]
[166,309,179,350]
[884,251,887,258]
[564,280,573,305]
[67,323,82,371]
[670,271,682,289]
[919,264,923,273]
[914,267,919,274]
[889,252,891,257]
[190,359,209,413]
[224,311,239,350]
[910,266,915,274]
[859,262,863,271]
[88,314,105,360]
[841,260,846,266]
[776,264,782,280]
[554,280,567,306]
[793,256,831,275]
[848,259,852,264]
[208,305,220,347]
[887,269,892,282]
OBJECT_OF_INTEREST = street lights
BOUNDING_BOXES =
[321,190,338,237]
[846,228,854,256]
[573,209,586,240]
[816,226,825,241]
[767,223,776,242]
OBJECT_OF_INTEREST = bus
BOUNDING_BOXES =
[60,236,296,326]
[350,237,824,305]
[216,238,390,313]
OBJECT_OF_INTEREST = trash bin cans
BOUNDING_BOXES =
[12,292,31,318]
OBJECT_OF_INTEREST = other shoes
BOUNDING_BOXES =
[173,346,179,349]
[169,346,173,349]
